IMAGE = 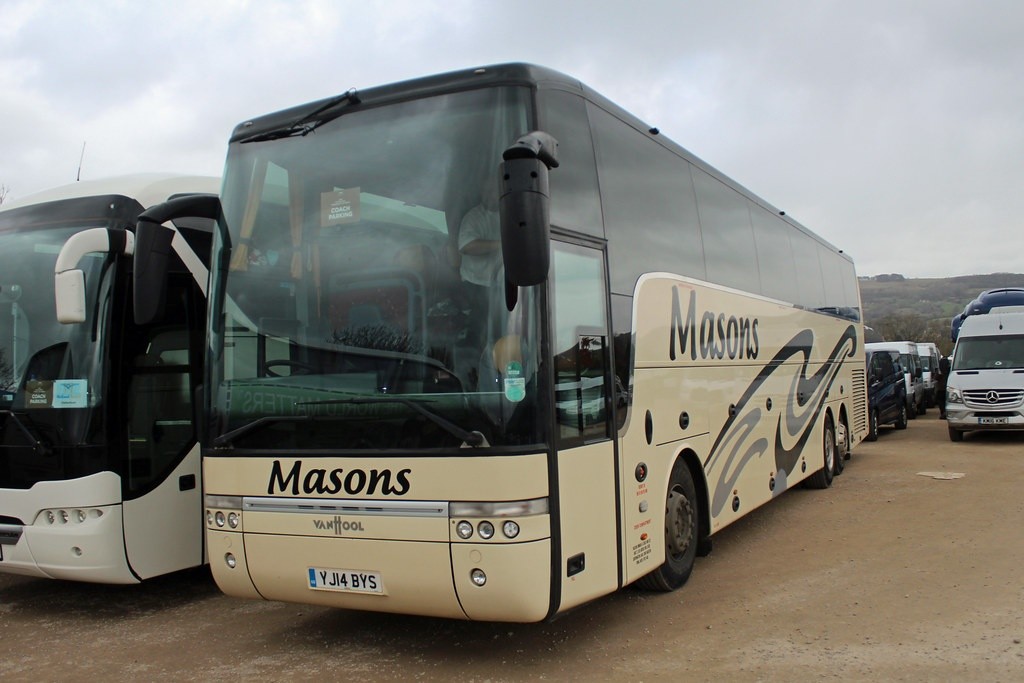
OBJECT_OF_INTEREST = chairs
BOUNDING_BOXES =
[320,267,429,388]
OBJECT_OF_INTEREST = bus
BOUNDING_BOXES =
[2,171,447,589]
[131,61,870,628]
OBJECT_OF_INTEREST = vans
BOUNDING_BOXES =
[863,341,927,419]
[915,342,943,408]
[863,347,909,441]
[946,305,1024,444]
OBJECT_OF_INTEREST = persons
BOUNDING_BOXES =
[935,357,951,419]
[458,175,500,349]
